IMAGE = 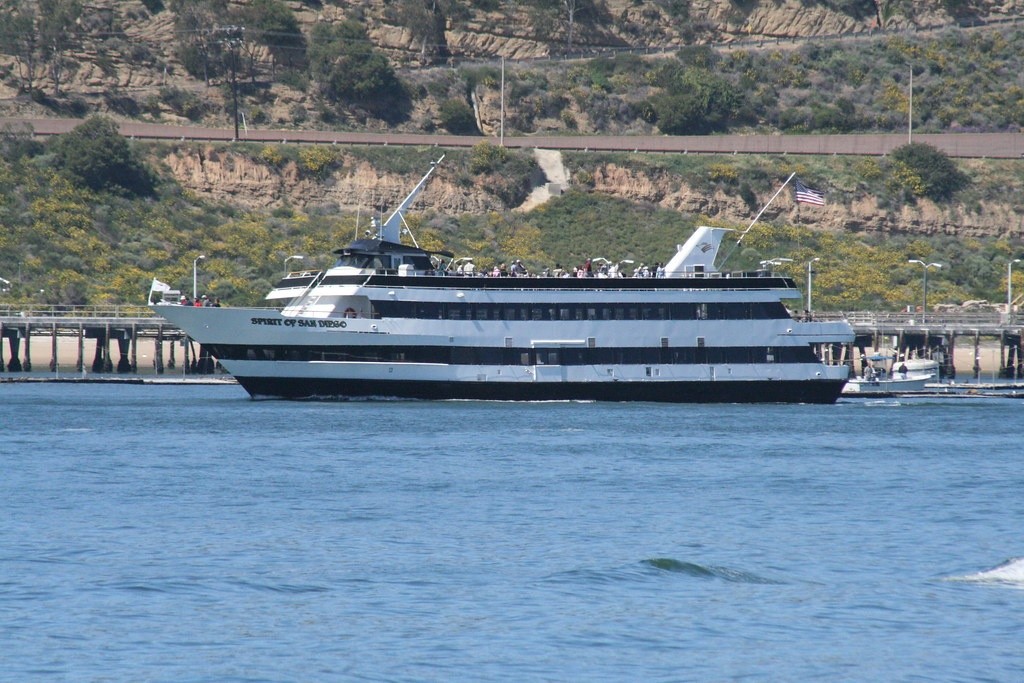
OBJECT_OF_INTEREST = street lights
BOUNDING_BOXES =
[284,255,304,277]
[908,258,944,324]
[806,257,821,313]
[193,255,206,297]
[1008,259,1021,325]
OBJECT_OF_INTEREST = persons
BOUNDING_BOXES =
[431,258,665,278]
[786,305,908,382]
[180,294,221,307]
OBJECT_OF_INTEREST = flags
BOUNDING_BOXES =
[795,180,825,206]
[152,280,171,293]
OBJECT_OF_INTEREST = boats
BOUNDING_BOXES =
[889,345,940,380]
[840,355,936,395]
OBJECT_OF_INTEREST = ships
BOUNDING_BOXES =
[145,153,858,401]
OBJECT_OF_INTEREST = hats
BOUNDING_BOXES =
[517,260,520,263]
[180,296,186,300]
[511,260,515,264]
[640,263,644,267]
[643,266,649,270]
[202,295,207,298]
